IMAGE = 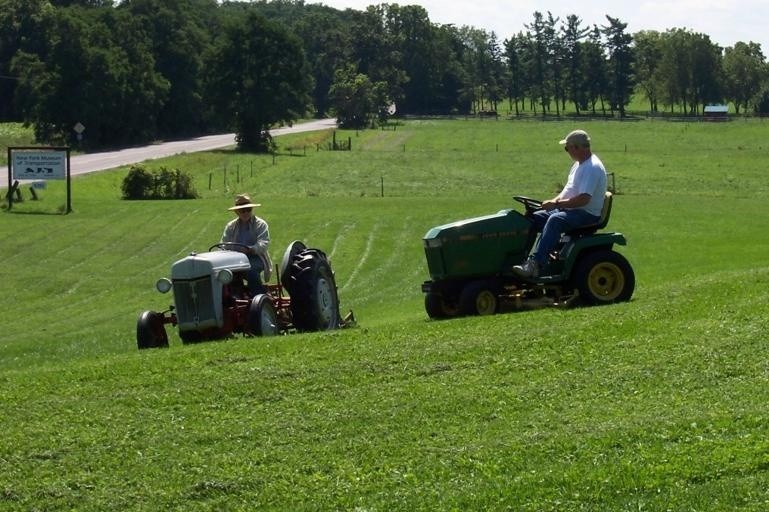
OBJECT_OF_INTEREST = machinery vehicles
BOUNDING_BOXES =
[418,168,635,325]
[133,239,355,351]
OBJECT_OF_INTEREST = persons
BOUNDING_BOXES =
[218,194,273,305]
[512,130,607,278]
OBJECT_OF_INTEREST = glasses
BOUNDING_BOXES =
[238,209,252,213]
[564,147,570,152]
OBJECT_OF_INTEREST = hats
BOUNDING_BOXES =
[227,193,260,212]
[561,131,590,146]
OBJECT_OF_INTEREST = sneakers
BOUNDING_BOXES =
[513,256,539,277]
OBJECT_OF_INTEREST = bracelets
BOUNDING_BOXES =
[556,198,560,206]
